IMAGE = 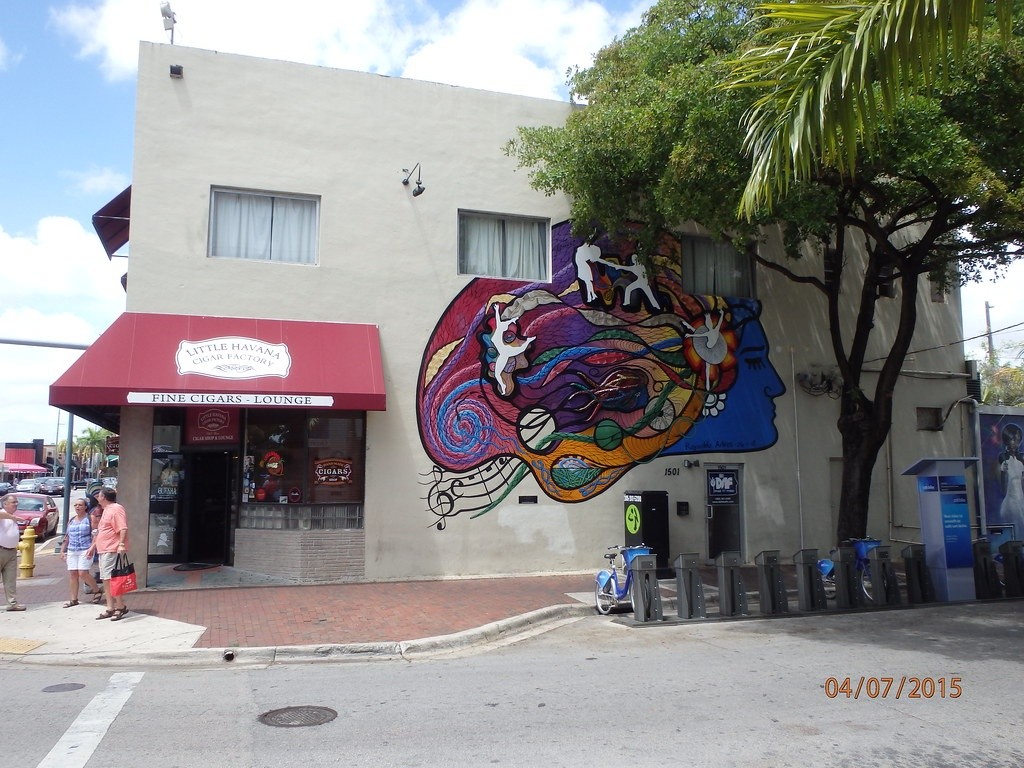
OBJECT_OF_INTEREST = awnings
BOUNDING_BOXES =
[91,185,132,261]
[3,463,51,474]
[48,313,387,436]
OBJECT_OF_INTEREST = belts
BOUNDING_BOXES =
[0,545,15,550]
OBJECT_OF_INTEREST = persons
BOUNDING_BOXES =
[0,495,26,611]
[59,484,128,621]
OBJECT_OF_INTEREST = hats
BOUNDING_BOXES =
[87,481,104,496]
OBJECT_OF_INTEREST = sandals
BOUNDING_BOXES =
[96,608,115,619]
[62,598,79,608]
[110,605,129,621]
[92,586,103,604]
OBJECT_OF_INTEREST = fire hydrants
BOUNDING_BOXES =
[18,525,38,577]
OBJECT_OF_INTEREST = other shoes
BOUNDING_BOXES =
[83,583,91,594]
[6,606,26,611]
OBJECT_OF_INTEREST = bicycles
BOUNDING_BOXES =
[594,543,654,617]
[819,536,888,602]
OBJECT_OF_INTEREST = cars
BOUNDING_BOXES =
[39,478,66,497]
[0,483,16,497]
[0,493,60,542]
[70,478,93,490]
[16,479,42,493]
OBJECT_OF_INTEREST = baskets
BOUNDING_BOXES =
[852,539,882,561]
[622,548,649,570]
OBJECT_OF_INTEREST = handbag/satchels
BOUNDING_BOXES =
[109,552,138,596]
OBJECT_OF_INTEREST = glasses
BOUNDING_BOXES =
[73,503,85,506]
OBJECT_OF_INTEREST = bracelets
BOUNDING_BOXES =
[118,543,125,546]
